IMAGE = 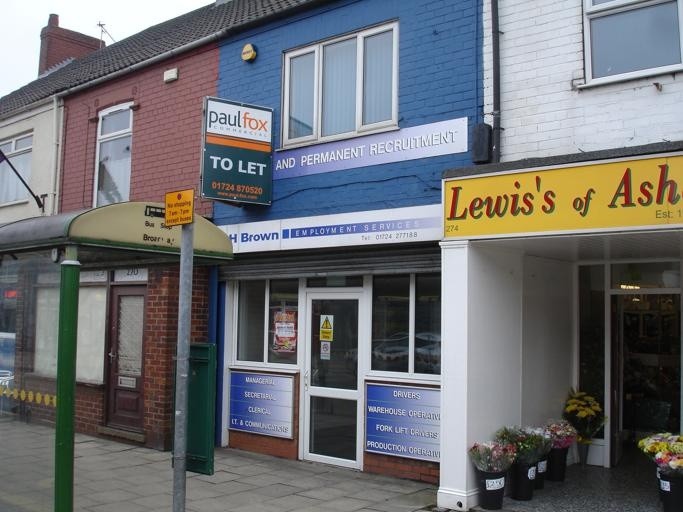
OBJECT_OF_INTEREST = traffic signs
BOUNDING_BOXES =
[162,190,193,226]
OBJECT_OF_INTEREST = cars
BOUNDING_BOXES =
[344,328,440,373]
[0,370,13,393]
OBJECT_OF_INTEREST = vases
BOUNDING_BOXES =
[658,467,683,511]
[508,463,537,500]
[656,463,661,488]
[534,454,547,490]
[577,435,589,467]
[549,444,570,481]
[478,469,509,510]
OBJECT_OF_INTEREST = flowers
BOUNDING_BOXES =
[644,435,682,470]
[561,383,607,439]
[467,441,517,474]
[498,424,539,465]
[523,426,553,460]
[635,430,669,467]
[542,420,576,448]
